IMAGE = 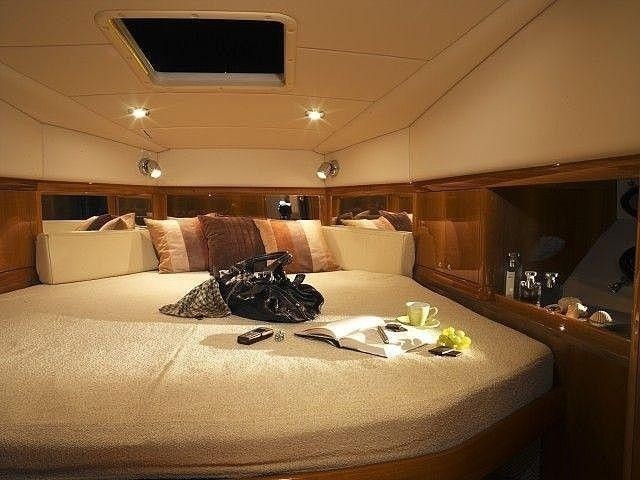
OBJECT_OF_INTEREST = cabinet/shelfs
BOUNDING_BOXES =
[415,189,537,290]
[418,267,633,466]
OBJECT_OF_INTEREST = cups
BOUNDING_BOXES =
[406,302,437,324]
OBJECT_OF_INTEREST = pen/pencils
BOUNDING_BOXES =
[377,326,389,344]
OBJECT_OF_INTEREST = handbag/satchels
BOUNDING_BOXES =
[217,266,324,323]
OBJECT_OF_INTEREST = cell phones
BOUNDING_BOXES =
[238,327,274,344]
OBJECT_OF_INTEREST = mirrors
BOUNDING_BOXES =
[40,194,154,235]
[329,192,415,232]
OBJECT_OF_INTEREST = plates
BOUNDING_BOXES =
[397,315,440,329]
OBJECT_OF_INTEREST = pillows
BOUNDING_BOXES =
[67,210,137,233]
[142,216,212,274]
[334,207,412,232]
[195,213,270,274]
[252,219,343,275]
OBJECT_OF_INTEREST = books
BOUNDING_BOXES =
[294,315,436,359]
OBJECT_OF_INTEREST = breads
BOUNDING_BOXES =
[559,297,612,323]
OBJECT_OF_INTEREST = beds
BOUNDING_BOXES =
[0,209,554,480]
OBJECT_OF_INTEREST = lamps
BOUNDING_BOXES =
[315,159,340,181]
[139,158,163,180]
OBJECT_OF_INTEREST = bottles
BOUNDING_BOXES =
[522,272,541,303]
[544,272,561,307]
[505,248,520,298]
